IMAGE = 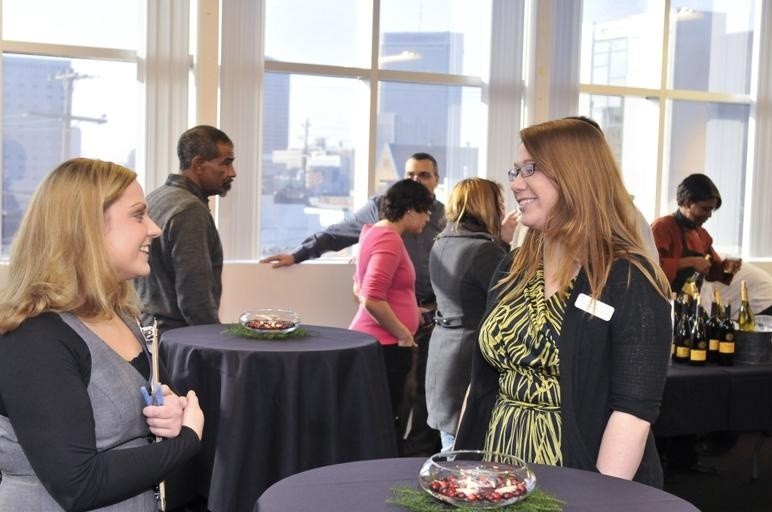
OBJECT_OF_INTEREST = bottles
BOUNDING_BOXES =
[670,280,757,368]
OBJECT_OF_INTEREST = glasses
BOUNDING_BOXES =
[507,164,536,182]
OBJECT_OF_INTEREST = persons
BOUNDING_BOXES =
[257,151,447,442]
[125,124,236,343]
[346,181,431,458]
[0,157,205,511]
[648,174,742,301]
[424,179,519,466]
[451,117,671,491]
